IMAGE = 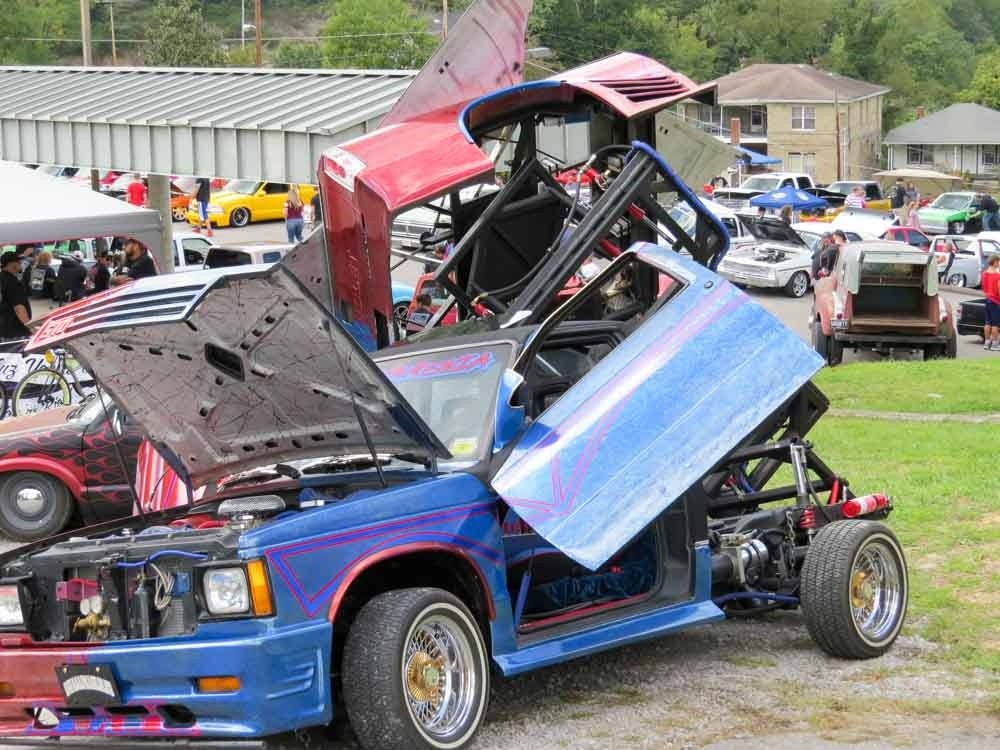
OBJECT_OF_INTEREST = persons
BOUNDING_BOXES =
[779,206,793,226]
[283,190,305,245]
[310,186,322,231]
[127,172,145,208]
[191,178,213,237]
[16,238,158,303]
[844,185,866,209]
[936,243,954,284]
[0,251,33,339]
[888,177,921,229]
[708,175,729,194]
[975,192,1000,232]
[981,254,1000,351]
[423,240,456,299]
[758,206,766,217]
[811,229,848,315]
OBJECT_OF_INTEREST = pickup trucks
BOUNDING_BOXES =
[712,169,832,219]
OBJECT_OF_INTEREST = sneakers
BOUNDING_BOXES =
[984,344,991,350]
[991,344,1000,350]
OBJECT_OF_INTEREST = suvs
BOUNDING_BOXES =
[808,238,957,366]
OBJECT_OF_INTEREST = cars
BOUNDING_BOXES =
[655,193,741,259]
[813,179,1000,342]
[0,0,728,542]
[716,211,900,299]
[0,139,911,750]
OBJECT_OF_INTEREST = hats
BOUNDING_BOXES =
[0,251,25,268]
[72,251,83,261]
[95,250,116,257]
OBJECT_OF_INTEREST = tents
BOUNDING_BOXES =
[735,144,784,186]
[0,161,167,274]
[749,185,829,224]
[871,169,961,200]
